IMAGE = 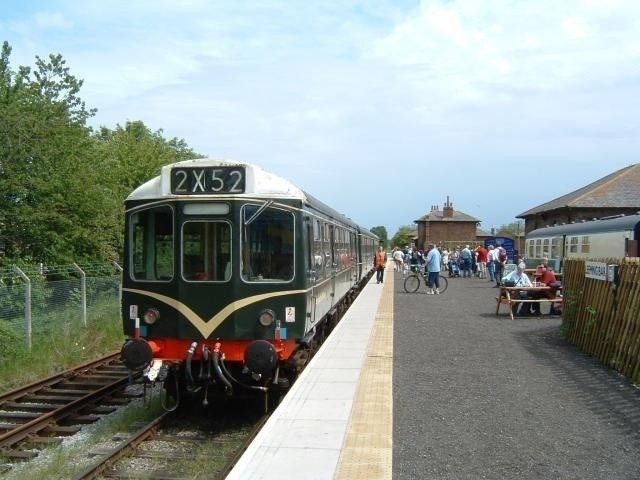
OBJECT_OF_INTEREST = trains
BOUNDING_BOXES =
[119,158,382,417]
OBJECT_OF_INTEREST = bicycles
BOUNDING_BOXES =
[403,261,448,294]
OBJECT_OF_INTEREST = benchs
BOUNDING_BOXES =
[511,298,563,306]
[496,296,512,304]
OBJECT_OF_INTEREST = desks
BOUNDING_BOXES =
[496,285,562,320]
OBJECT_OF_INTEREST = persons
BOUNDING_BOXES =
[532,263,556,315]
[374,247,387,284]
[502,262,533,316]
[257,236,293,280]
[392,243,507,295]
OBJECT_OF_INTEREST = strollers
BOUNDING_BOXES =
[447,259,462,278]
